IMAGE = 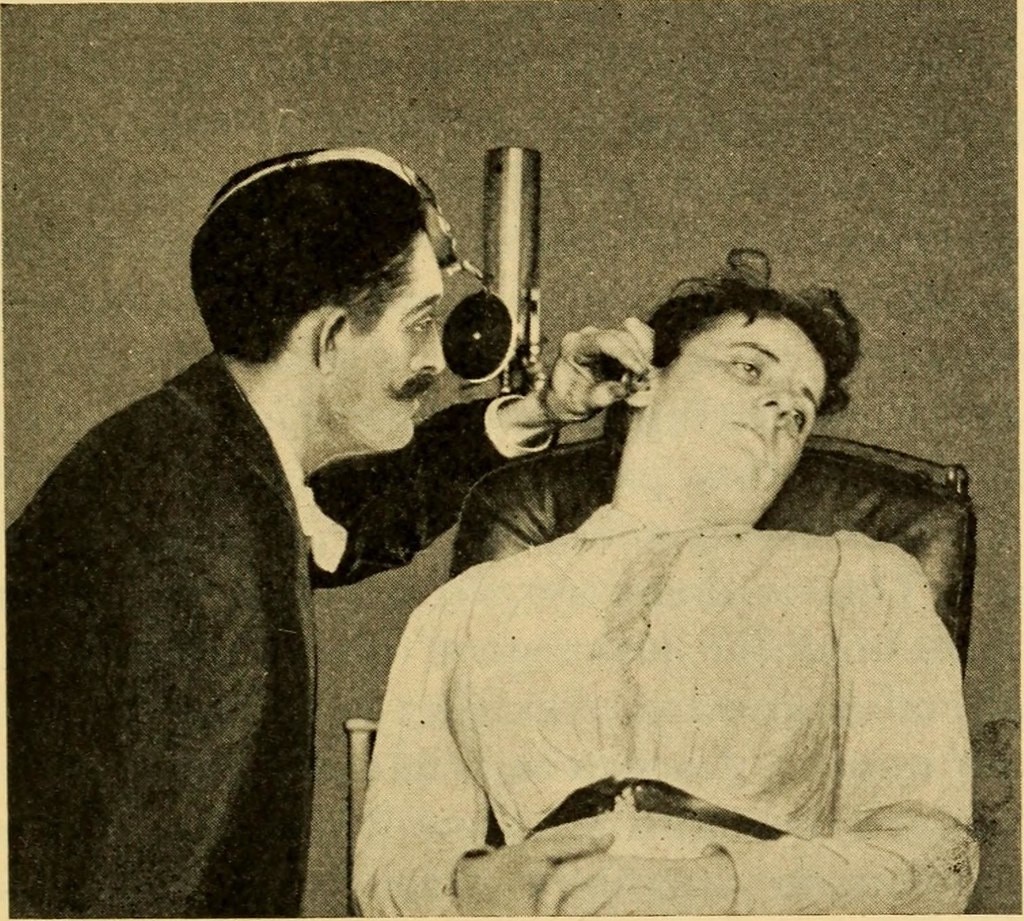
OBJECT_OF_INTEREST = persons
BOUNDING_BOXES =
[352,248,979,916]
[6,146,656,919]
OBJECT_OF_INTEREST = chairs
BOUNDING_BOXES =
[344,426,977,900]
